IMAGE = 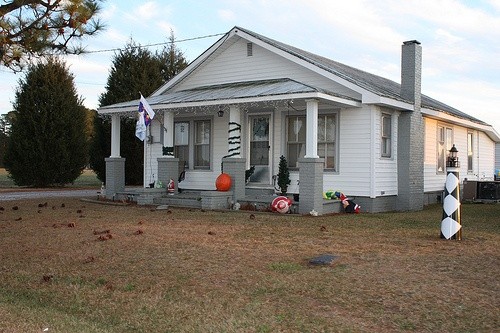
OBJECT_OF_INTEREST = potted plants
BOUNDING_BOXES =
[276,155,291,196]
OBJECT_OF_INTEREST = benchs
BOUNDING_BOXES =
[176,171,223,193]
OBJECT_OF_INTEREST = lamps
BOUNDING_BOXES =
[215,104,225,119]
[446,144,459,168]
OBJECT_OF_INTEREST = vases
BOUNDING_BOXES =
[292,193,300,202]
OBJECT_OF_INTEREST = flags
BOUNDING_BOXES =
[134,94,156,141]
